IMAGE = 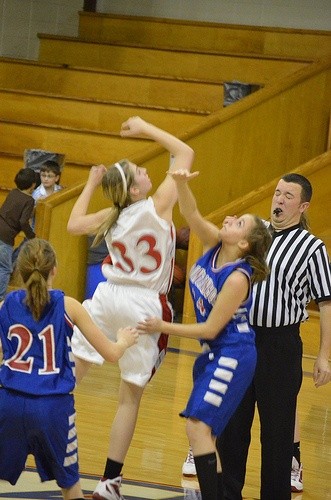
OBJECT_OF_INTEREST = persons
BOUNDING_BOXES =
[0,117,331,500]
[0,239,139,500]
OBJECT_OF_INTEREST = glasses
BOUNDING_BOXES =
[40,173,57,178]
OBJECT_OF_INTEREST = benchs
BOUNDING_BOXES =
[0,10,331,292]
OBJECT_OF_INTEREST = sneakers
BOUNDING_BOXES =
[91,474,130,500]
[291,456,305,492]
[181,445,198,477]
